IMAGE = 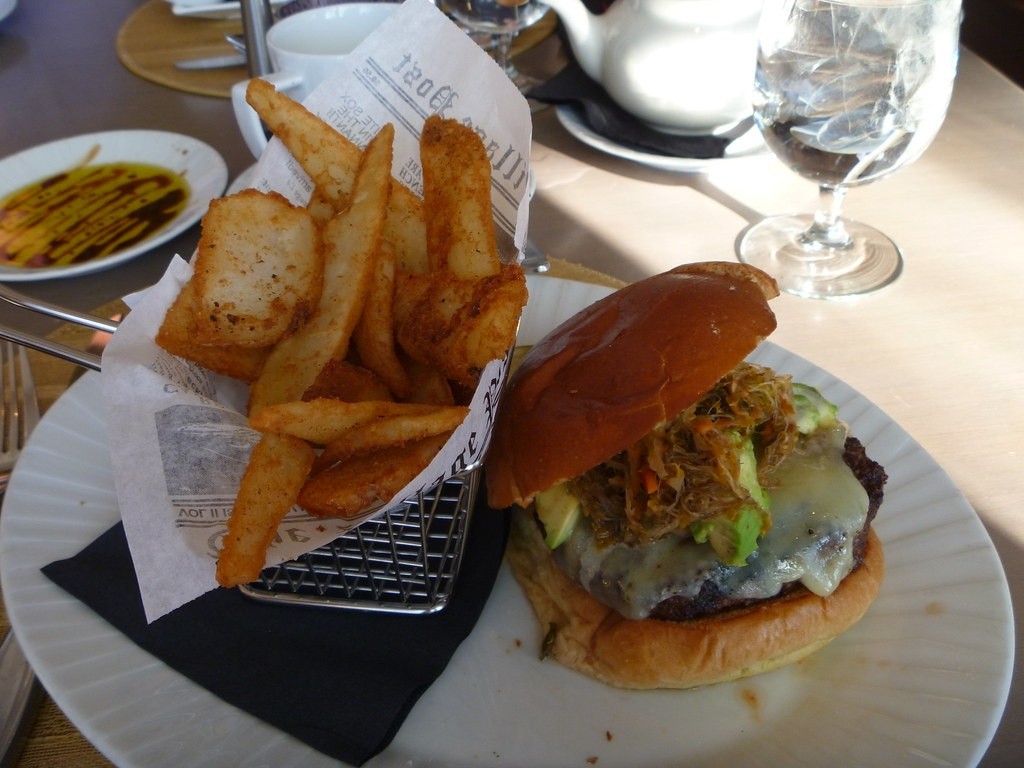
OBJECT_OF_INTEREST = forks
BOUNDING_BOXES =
[0,337,44,655]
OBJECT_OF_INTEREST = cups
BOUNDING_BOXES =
[230,1,411,164]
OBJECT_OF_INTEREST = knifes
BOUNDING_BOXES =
[174,53,248,74]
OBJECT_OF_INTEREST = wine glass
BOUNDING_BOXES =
[437,0,552,117]
[734,0,965,301]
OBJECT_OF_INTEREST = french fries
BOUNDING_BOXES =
[155,78,529,589]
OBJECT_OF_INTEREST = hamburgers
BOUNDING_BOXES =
[484,261,890,691]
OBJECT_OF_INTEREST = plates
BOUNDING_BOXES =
[552,91,781,174]
[1,129,228,282]
[3,259,1021,768]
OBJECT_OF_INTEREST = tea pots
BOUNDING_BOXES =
[536,0,795,137]
